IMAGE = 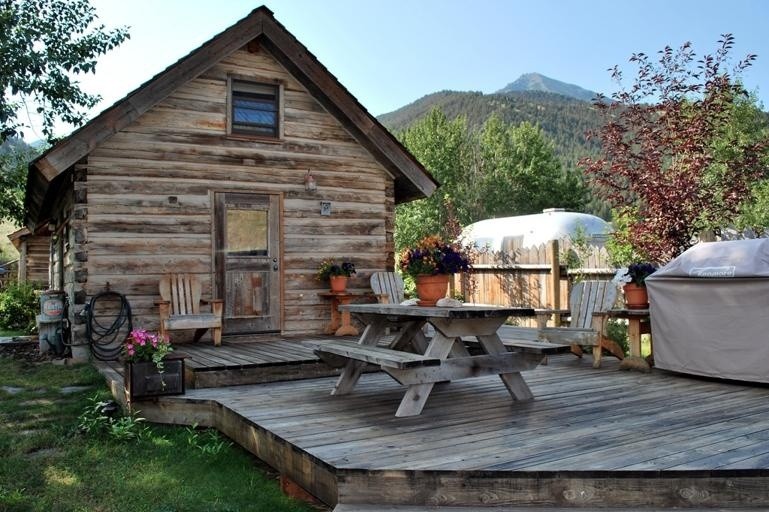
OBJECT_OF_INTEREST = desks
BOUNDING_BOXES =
[331,303,537,418]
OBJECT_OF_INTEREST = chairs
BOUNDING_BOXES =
[370,272,408,304]
[539,280,625,368]
[152,273,226,347]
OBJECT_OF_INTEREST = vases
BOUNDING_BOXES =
[117,352,193,404]
[414,274,450,305]
[329,276,349,292]
[622,282,648,308]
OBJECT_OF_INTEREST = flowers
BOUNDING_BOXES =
[314,258,357,280]
[398,237,471,274]
[118,325,175,393]
[624,264,654,287]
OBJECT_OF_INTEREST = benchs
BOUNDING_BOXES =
[461,336,573,402]
[300,336,442,418]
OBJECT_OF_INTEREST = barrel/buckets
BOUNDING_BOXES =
[40,294,64,322]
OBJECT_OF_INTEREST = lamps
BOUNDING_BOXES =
[304,167,316,192]
